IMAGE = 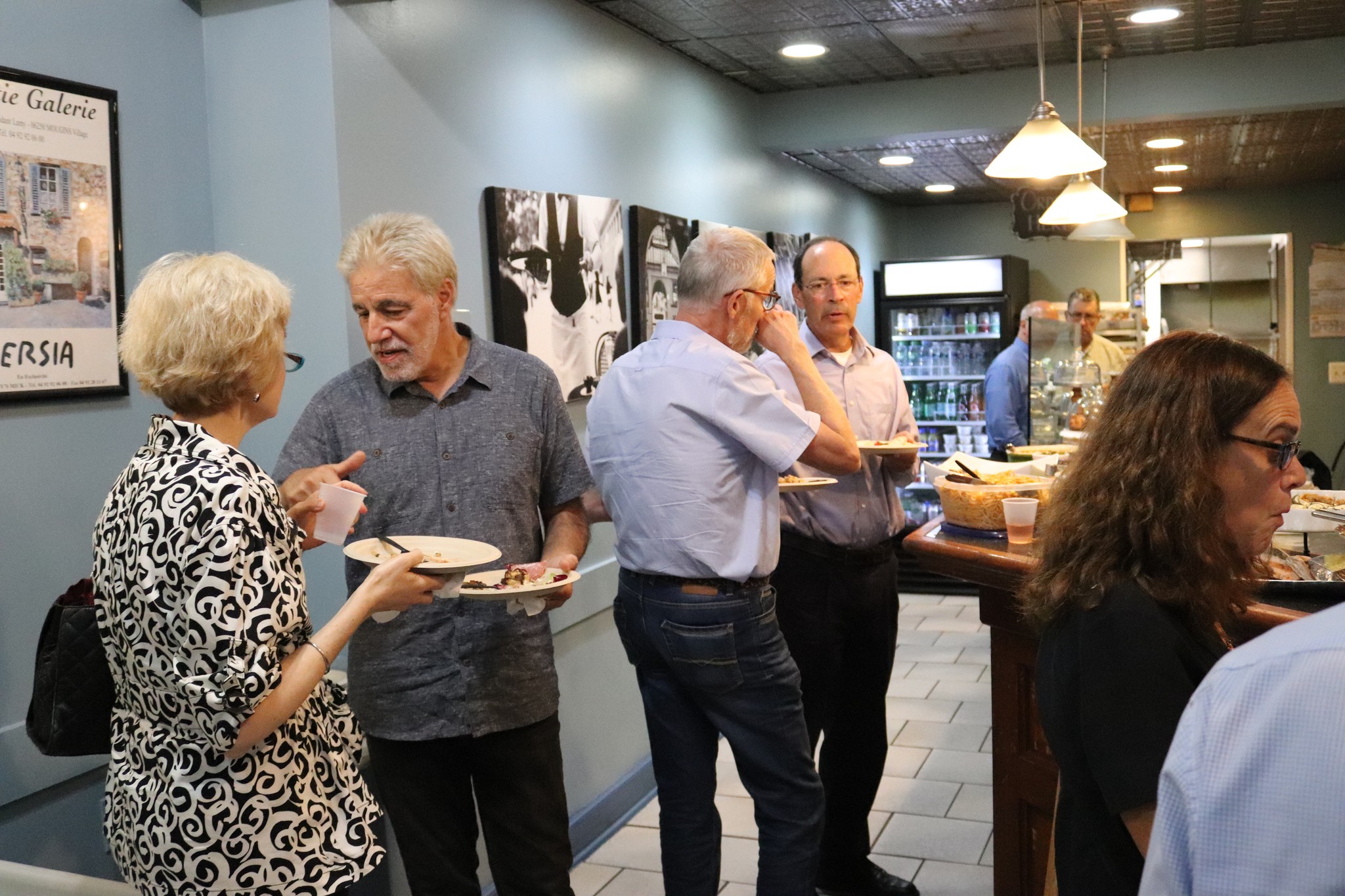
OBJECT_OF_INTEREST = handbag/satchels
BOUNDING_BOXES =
[23,581,116,757]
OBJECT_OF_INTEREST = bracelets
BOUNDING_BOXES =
[301,640,330,675]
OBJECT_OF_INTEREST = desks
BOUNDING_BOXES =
[903,491,1345,896]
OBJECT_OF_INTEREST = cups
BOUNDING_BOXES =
[943,425,989,453]
[1001,498,1039,544]
[312,482,368,546]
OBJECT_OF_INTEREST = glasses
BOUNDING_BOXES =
[724,289,782,311]
[278,349,305,373]
[796,275,859,298]
[1220,431,1302,470]
[1068,310,1100,324]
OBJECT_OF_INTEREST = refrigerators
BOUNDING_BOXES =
[876,253,1029,594]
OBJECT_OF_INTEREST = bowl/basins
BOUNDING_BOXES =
[1005,444,1079,462]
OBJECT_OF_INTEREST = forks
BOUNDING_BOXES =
[371,532,429,564]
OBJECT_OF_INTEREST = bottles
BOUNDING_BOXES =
[901,491,942,525]
[919,427,947,452]
[909,381,985,420]
[896,341,986,375]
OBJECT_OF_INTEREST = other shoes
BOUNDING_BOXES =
[813,853,920,896]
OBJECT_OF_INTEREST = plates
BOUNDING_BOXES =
[343,535,502,574]
[857,440,928,454]
[459,567,581,600]
[778,477,839,492]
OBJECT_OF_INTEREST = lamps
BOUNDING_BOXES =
[982,0,1139,242]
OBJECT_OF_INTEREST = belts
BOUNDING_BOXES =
[781,530,894,568]
[622,569,771,590]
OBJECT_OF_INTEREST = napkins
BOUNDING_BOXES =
[504,596,549,620]
[370,574,463,625]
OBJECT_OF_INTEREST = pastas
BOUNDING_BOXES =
[937,469,1049,529]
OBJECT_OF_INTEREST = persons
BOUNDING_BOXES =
[984,301,1061,462]
[269,212,595,896]
[1013,329,1307,896]
[584,228,862,896]
[750,237,921,896]
[1042,287,1128,388]
[90,250,447,896]
[1137,599,1345,896]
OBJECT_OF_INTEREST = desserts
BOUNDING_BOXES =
[1068,414,1088,431]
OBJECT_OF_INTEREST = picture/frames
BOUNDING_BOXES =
[0,66,129,410]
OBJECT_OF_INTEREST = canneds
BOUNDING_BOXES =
[955,311,1000,335]
